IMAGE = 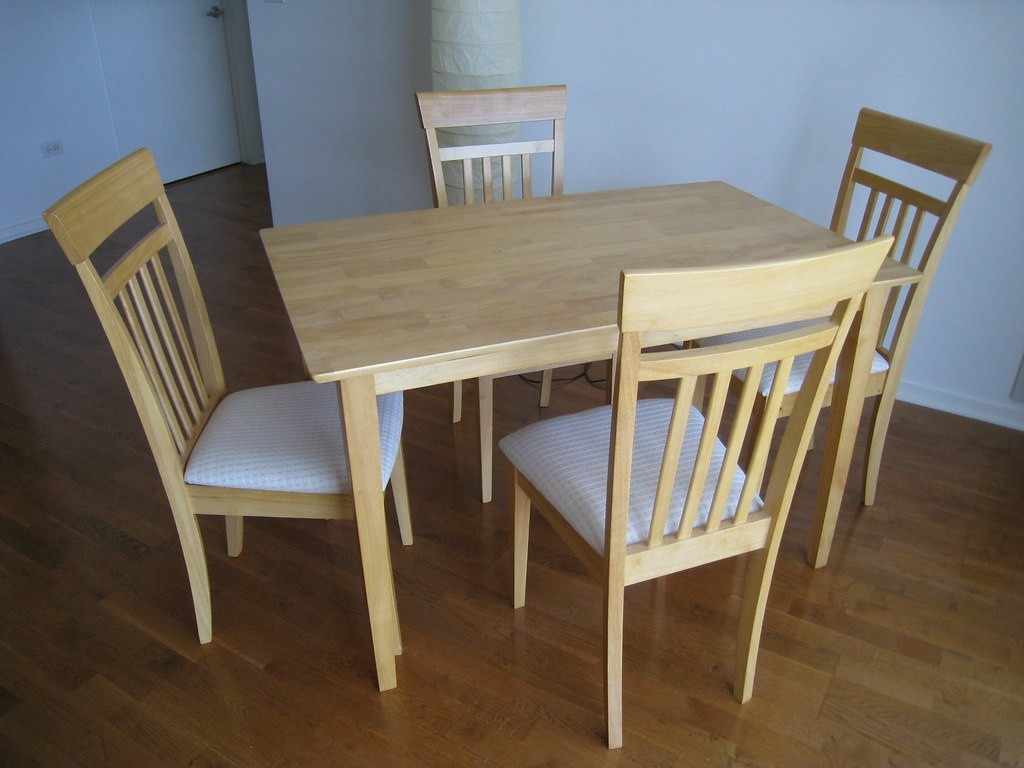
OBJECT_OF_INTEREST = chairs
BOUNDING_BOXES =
[497,235,896,749]
[42,147,414,656]
[692,104,992,507]
[412,84,569,424]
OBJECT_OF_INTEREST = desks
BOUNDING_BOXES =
[258,178,925,693]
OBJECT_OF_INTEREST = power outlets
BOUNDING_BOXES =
[41,139,65,159]
